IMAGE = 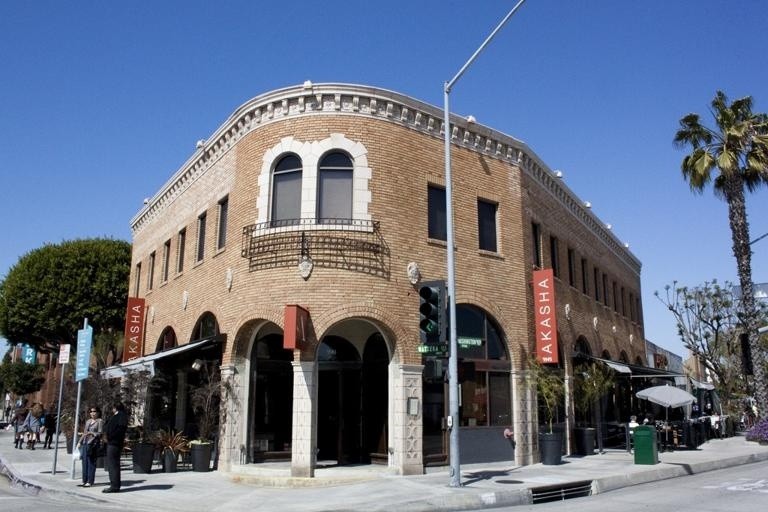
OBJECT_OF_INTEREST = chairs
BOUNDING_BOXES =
[626,420,681,453]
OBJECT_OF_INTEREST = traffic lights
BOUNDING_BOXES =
[418,280,446,345]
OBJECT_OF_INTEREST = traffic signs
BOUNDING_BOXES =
[417,345,448,354]
[456,336,482,349]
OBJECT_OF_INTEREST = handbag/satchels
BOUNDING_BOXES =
[87,438,101,457]
[22,425,28,432]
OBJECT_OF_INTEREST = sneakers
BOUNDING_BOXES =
[44,445,52,449]
[102,488,120,493]
[77,482,92,487]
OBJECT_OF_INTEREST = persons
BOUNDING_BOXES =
[76,406,105,487]
[628,413,665,437]
[101,401,128,493]
[5,399,56,450]
[698,410,719,430]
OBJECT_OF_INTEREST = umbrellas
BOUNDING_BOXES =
[636,384,698,441]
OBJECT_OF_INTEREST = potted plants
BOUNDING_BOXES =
[559,360,615,456]
[517,353,565,465]
[30,358,231,474]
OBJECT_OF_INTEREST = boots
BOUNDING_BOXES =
[14,438,36,450]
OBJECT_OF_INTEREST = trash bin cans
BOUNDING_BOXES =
[633,425,658,464]
